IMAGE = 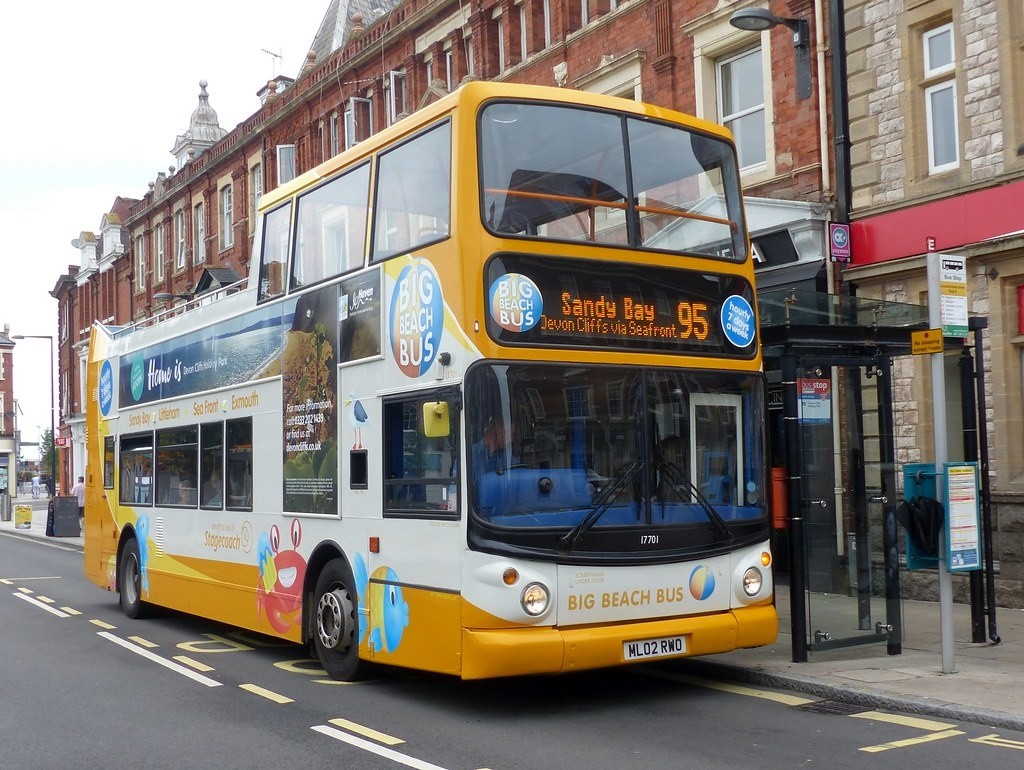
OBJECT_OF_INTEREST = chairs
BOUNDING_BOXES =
[416,227,448,245]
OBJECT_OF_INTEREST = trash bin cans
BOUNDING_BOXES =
[1,494,11,522]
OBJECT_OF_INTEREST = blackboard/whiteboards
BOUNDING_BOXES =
[46,501,55,536]
[53,496,81,537]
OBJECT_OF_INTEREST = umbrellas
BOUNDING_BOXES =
[892,469,945,559]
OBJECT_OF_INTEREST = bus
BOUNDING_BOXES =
[81,79,782,681]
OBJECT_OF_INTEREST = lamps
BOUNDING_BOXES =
[153,292,191,303]
[729,8,812,100]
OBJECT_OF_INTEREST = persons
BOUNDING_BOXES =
[45,475,53,498]
[32,474,41,499]
[445,414,522,514]
[207,469,223,505]
[70,476,85,533]
[177,480,194,505]
[18,471,25,495]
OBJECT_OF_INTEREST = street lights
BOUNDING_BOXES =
[11,334,59,497]
